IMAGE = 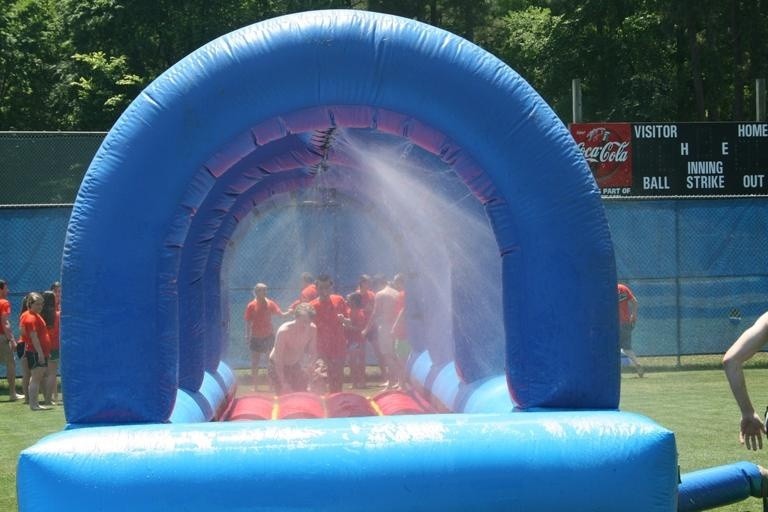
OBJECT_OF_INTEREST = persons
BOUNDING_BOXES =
[265,272,409,396]
[0,280,26,402]
[244,283,293,394]
[16,282,60,411]
[720,310,767,452]
[617,283,645,379]
